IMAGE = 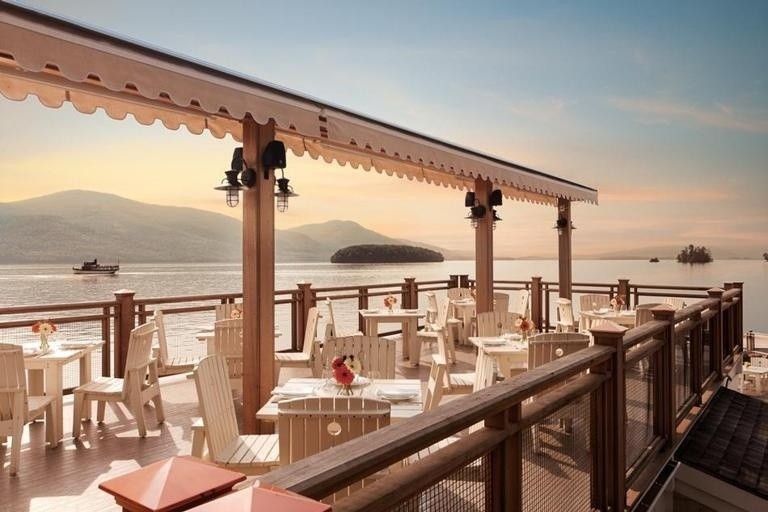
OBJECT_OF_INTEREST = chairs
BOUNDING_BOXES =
[752,357,764,366]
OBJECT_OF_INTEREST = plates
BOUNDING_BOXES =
[403,309,417,312]
[329,376,372,387]
[273,384,312,400]
[593,310,606,314]
[362,308,380,313]
[622,312,635,317]
[477,337,506,345]
[58,342,89,350]
[503,333,522,340]
[374,387,419,402]
[21,348,36,357]
[453,299,467,303]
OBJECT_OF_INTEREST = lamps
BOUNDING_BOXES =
[463,198,479,226]
[214,156,256,209]
[493,207,502,232]
[273,167,294,213]
[553,212,565,235]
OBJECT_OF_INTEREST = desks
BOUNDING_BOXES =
[741,366,767,396]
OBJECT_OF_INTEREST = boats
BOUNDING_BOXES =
[73,259,120,275]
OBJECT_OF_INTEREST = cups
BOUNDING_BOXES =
[620,305,627,313]
